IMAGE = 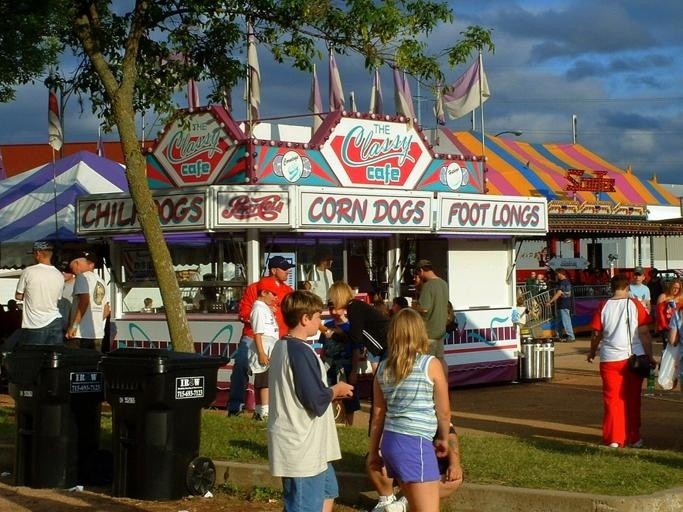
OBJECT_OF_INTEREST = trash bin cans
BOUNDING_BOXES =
[7,346,98,489]
[520,336,555,382]
[105,348,224,495]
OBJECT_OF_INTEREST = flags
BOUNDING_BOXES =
[242,18,263,134]
[440,47,490,122]
[307,42,447,137]
[185,72,200,114]
[43,66,64,155]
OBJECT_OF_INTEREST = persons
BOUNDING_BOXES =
[535,247,546,267]
[584,273,657,448]
[363,420,465,510]
[368,307,451,511]
[516,265,683,397]
[54,257,74,342]
[248,277,281,421]
[79,248,110,341]
[3,300,22,339]
[63,247,110,352]
[297,246,451,427]
[142,297,152,309]
[265,289,356,512]
[227,255,295,419]
[14,238,68,347]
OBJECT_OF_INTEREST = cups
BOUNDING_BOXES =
[404,297,413,307]
[383,299,389,309]
[667,299,677,309]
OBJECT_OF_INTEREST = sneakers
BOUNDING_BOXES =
[383,496,409,512]
[369,493,398,512]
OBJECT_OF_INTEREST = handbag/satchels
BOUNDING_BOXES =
[446,301,458,335]
[626,297,650,378]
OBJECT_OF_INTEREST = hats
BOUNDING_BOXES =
[26,239,55,255]
[633,267,643,276]
[256,276,282,295]
[268,255,296,270]
[412,259,433,275]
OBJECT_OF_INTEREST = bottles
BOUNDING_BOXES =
[646,367,655,395]
